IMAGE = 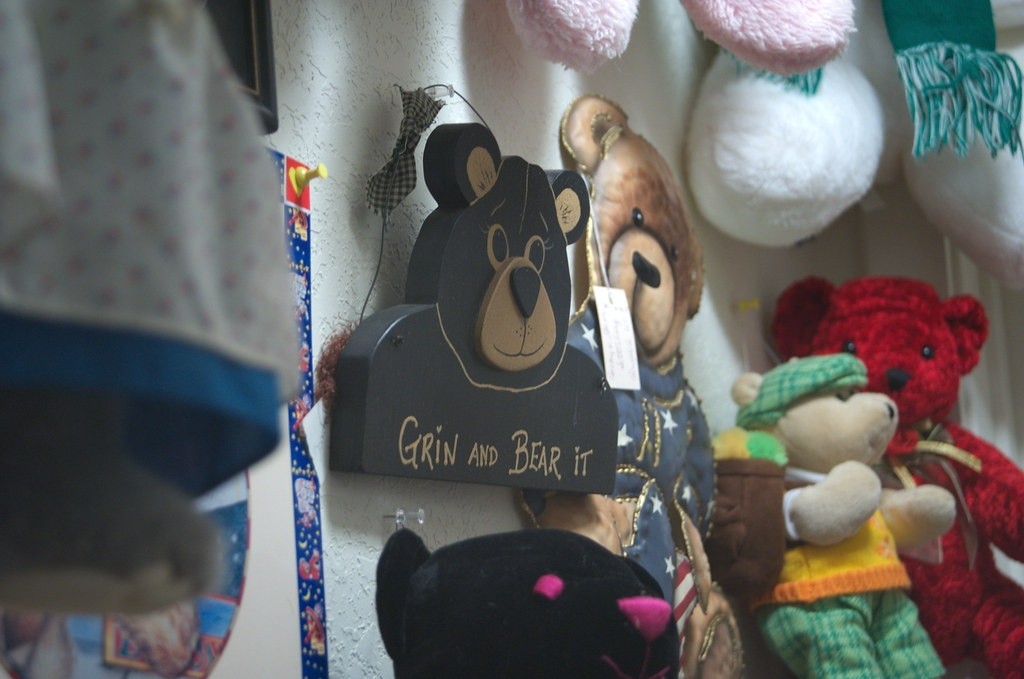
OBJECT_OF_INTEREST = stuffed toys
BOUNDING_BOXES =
[730,354,946,679]
[375,527,680,679]
[768,276,1024,679]
[685,0,1024,287]
[508,0,858,76]
